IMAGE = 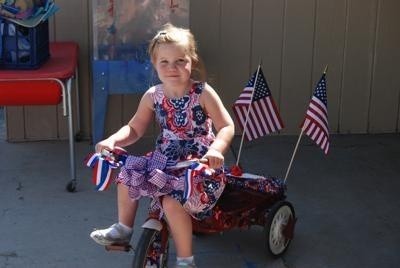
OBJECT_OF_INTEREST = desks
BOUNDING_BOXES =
[0,41,82,191]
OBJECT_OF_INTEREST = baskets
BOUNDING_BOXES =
[1,20,50,70]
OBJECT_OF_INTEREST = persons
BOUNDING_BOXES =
[90,22,236,267]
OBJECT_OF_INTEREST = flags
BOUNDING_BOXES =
[297,73,332,155]
[232,65,284,144]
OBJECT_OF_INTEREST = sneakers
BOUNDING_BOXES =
[90,223,134,246]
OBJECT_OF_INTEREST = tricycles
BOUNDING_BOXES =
[95,146,298,268]
